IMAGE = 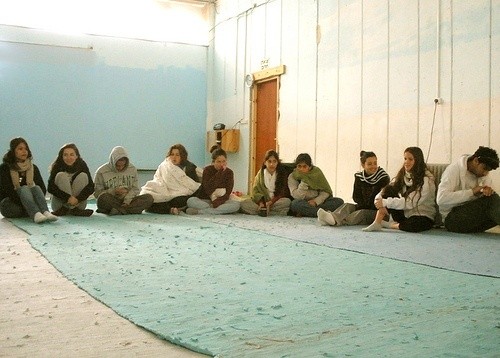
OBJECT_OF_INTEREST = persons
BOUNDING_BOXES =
[144,143,200,215]
[92,145,154,216]
[47,143,95,216]
[315,150,391,226]
[0,137,58,223]
[183,145,241,215]
[361,147,436,233]
[287,152,344,217]
[437,146,500,233]
[239,150,295,217]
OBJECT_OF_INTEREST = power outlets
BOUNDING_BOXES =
[433,98,440,103]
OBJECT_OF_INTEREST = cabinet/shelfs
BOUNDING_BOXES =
[207,129,240,152]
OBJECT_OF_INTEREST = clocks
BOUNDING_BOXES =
[244,73,254,88]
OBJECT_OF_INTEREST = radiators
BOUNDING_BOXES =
[425,163,451,223]
